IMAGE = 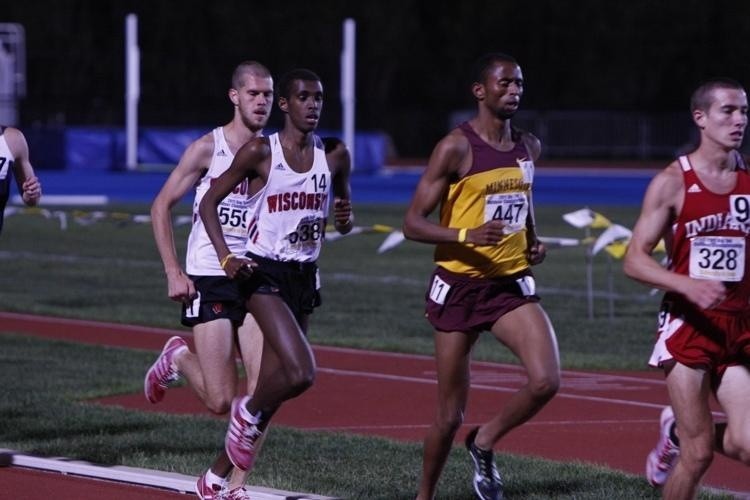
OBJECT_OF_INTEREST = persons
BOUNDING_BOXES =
[402,50,562,499]
[0,106,45,258]
[199,68,355,499]
[624,75,750,499]
[145,61,275,499]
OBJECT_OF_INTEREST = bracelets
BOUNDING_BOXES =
[221,253,236,269]
[459,228,466,246]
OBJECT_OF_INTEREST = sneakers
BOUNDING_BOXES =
[465,427,503,500]
[144,337,188,404]
[646,404,682,488]
[222,395,262,472]
[223,487,249,500]
[194,471,221,500]
